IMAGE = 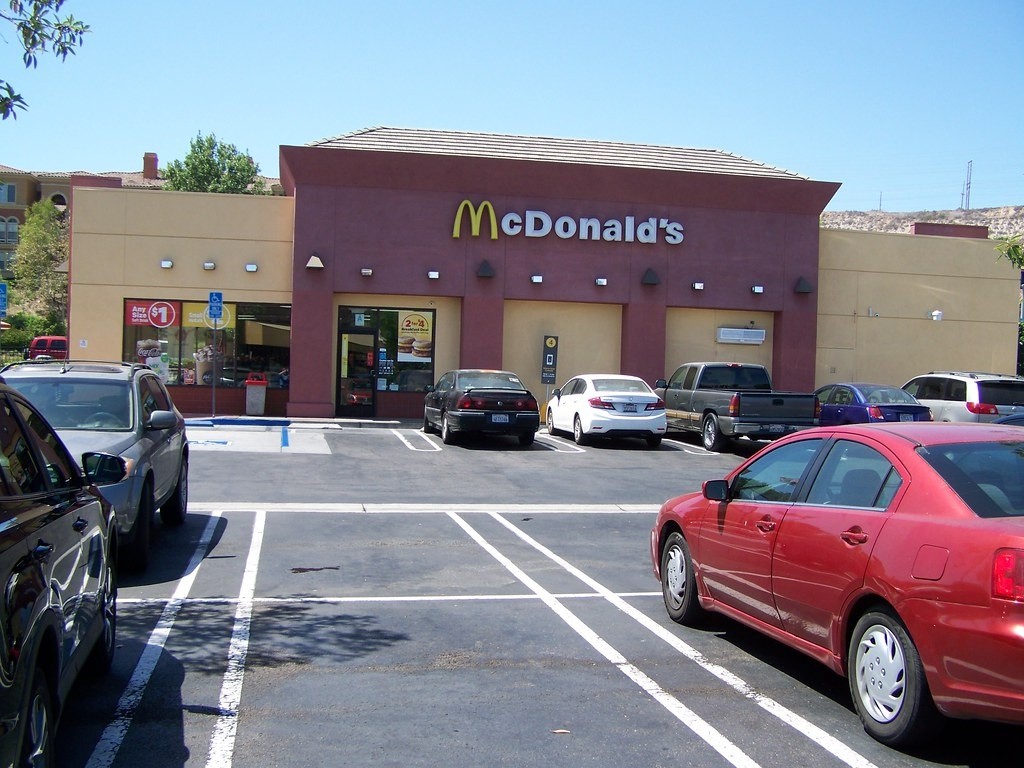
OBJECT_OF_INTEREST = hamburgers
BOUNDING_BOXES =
[412,341,431,356]
[398,337,415,352]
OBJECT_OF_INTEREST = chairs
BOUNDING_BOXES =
[629,387,638,392]
[967,471,1006,492]
[95,396,129,424]
[597,385,607,391]
[869,397,879,403]
[840,395,850,404]
[841,469,888,508]
[888,396,897,402]
[951,388,963,400]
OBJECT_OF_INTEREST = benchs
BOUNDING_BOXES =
[58,405,97,423]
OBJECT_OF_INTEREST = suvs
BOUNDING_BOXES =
[900,370,1024,423]
[28,336,69,360]
[0,361,189,559]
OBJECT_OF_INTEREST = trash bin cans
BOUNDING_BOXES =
[245,372,269,416]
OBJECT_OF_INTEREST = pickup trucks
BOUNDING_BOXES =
[651,361,821,452]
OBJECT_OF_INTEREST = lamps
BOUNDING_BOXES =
[477,261,494,278]
[306,252,324,268]
[641,269,661,285]
[794,278,814,293]
[927,310,943,321]
[926,384,943,399]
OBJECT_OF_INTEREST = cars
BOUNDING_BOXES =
[347,377,373,406]
[812,383,935,425]
[424,368,541,446]
[648,421,1024,768]
[544,373,667,447]
[0,381,121,768]
